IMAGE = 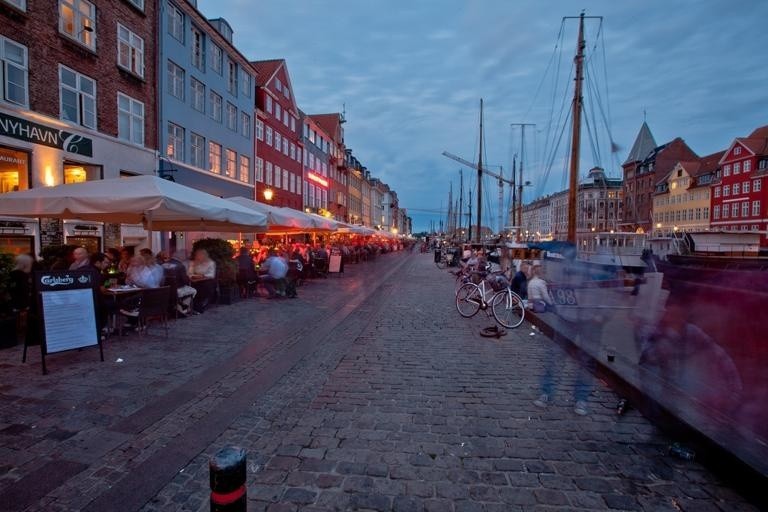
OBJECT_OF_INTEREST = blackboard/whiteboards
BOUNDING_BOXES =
[36,269,101,354]
[328,254,342,273]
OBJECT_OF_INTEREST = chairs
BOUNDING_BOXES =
[1,220,409,378]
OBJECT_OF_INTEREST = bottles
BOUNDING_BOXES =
[109,262,119,289]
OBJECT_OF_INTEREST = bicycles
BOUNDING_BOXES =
[431,247,526,329]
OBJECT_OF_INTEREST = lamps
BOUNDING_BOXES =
[76,24,94,38]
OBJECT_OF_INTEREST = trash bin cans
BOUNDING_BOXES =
[435,249,441,262]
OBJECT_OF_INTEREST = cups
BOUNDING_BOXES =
[104,280,110,289]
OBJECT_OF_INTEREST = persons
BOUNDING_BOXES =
[532,228,626,415]
[420,236,558,315]
